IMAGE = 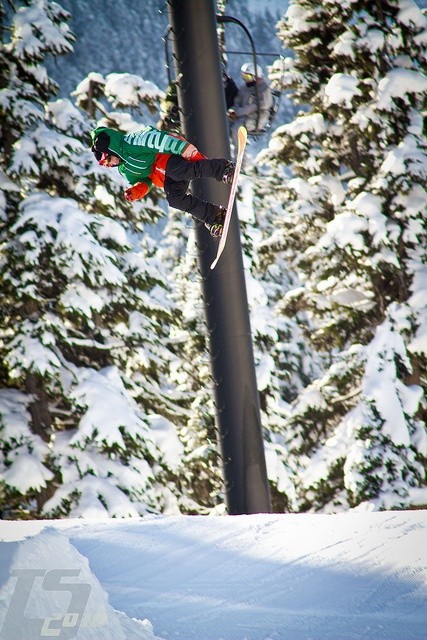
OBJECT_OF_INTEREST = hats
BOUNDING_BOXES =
[91,143,105,165]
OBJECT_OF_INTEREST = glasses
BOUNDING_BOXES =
[105,155,111,168]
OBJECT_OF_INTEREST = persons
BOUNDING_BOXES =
[92,127,236,240]
[156,81,180,133]
[225,63,271,164]
[221,54,237,109]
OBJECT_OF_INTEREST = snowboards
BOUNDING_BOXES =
[210,126,248,271]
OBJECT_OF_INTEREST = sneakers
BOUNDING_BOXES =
[223,160,236,183]
[210,206,226,237]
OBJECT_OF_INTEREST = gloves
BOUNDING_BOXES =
[124,188,138,202]
[189,151,208,161]
